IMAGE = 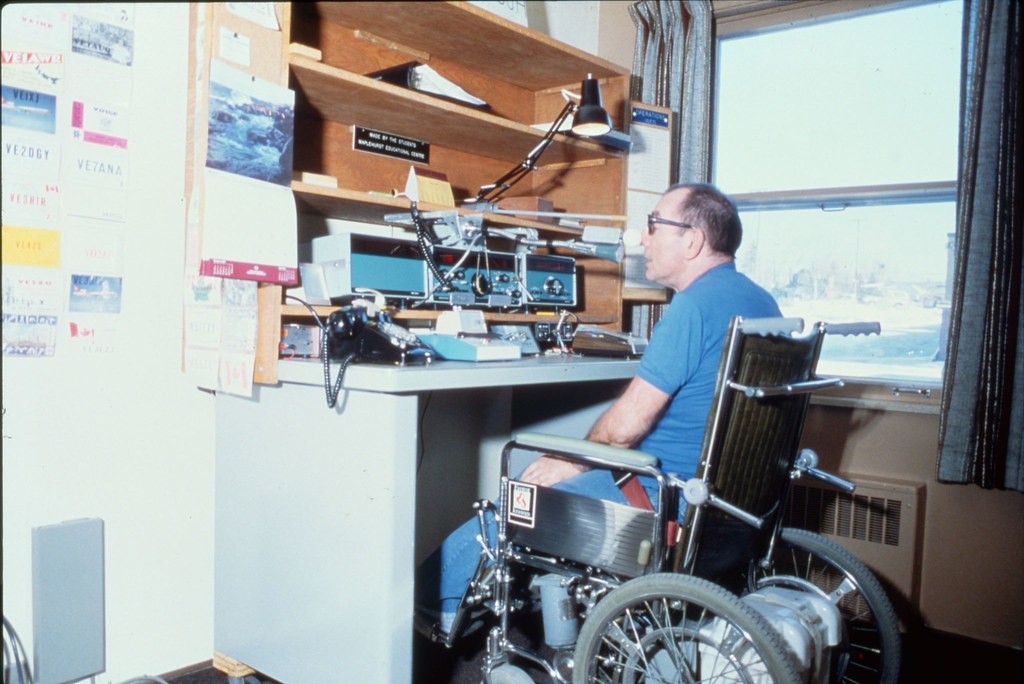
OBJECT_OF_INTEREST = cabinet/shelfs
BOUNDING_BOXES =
[250,0,633,383]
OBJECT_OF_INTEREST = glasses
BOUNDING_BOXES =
[648,214,692,236]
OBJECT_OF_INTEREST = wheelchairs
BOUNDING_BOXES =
[413,317,902,684]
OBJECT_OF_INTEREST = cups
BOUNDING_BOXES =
[532,575,578,645]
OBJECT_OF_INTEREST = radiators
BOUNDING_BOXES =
[803,469,928,636]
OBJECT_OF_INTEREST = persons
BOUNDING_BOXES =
[414,182,792,634]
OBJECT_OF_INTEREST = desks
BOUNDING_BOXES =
[211,356,640,684]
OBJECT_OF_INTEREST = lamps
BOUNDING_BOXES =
[455,73,613,211]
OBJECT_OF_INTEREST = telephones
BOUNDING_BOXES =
[320,306,436,368]
